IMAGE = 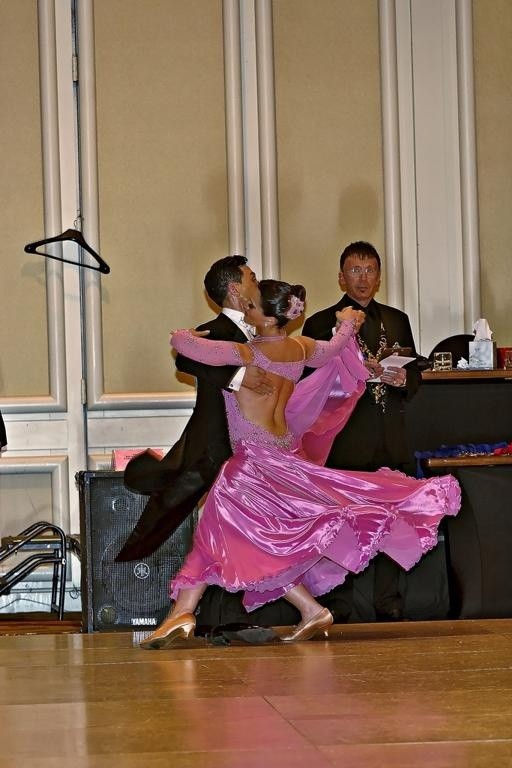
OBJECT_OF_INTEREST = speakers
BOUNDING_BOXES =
[79,469,198,634]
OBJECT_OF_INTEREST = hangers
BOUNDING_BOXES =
[23,219,111,275]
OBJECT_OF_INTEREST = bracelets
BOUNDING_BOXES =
[401,379,407,387]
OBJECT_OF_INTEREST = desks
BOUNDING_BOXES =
[403,368,511,620]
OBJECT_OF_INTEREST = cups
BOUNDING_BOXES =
[496,346,511,369]
[432,351,452,372]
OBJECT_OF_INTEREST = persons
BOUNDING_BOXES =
[116,256,366,641]
[140,279,461,648]
[300,241,422,476]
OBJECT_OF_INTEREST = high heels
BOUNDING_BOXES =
[280,607,333,640]
[140,613,197,650]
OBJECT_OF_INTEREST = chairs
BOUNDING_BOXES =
[428,334,475,369]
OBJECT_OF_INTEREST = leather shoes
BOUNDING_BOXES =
[212,622,278,645]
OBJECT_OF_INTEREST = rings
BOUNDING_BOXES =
[393,377,396,384]
